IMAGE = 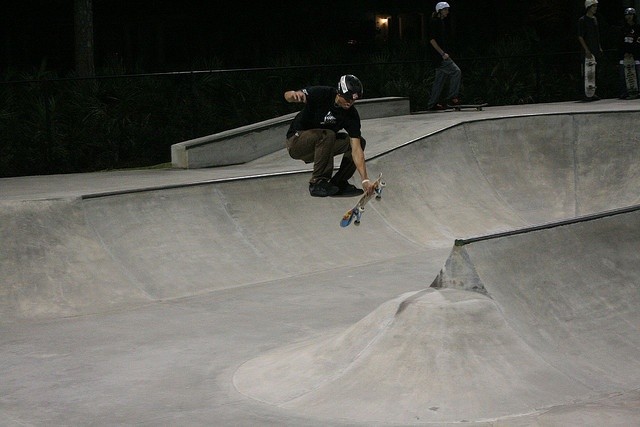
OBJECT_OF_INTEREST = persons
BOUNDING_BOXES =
[617,8,640,100]
[285,75,375,197]
[427,1,462,110]
[577,0,603,101]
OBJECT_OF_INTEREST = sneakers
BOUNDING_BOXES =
[448,99,462,106]
[331,174,364,197]
[309,180,340,197]
[428,103,446,111]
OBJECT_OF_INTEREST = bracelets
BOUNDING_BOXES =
[362,179,369,184]
[442,52,445,56]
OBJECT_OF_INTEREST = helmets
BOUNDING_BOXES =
[585,0,598,9]
[338,74,364,99]
[435,2,450,13]
[625,8,636,15]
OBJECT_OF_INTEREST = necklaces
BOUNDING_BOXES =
[334,98,341,107]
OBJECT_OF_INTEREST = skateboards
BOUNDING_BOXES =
[340,173,386,228]
[624,53,638,91]
[446,103,488,112]
[585,54,597,98]
